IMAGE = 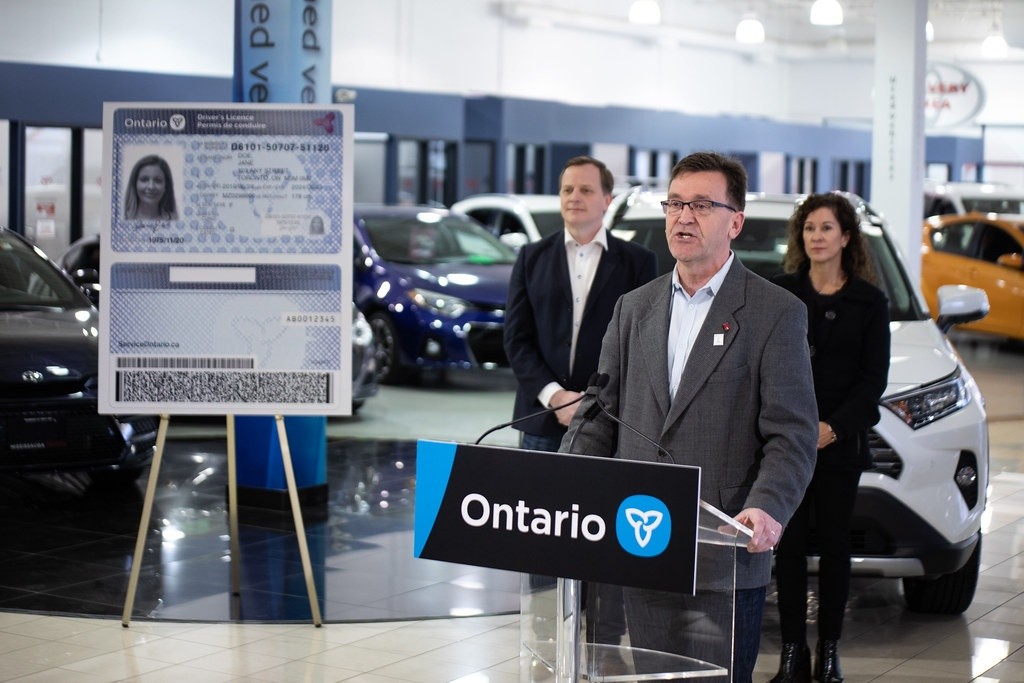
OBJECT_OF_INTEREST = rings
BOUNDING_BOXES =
[771,531,775,535]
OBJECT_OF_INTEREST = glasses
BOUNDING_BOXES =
[660,199,736,216]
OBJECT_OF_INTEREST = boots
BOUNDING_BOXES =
[814,639,843,683]
[770,642,813,683]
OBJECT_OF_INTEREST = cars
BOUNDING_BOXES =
[924,180,1023,238]
[1,227,163,490]
[31,232,376,421]
[449,195,565,259]
[922,212,1024,340]
[349,202,520,385]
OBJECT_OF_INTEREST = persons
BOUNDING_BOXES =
[504,156,656,680]
[558,153,820,683]
[765,193,890,683]
[124,155,179,221]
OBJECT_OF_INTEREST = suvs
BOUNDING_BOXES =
[604,185,990,619]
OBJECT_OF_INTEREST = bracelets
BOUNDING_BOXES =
[828,425,837,442]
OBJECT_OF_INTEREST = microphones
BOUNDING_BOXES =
[476,372,677,466]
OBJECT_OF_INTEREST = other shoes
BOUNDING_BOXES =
[586,647,628,675]
